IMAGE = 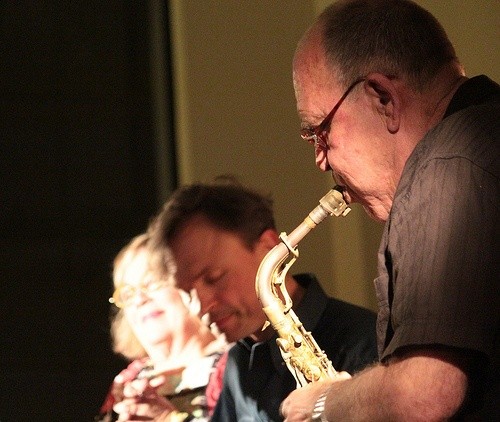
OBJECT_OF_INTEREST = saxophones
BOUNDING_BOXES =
[253,184,354,390]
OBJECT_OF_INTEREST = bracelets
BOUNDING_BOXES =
[311,391,328,422]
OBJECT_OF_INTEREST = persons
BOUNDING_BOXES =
[99,176,378,422]
[280,0,500,422]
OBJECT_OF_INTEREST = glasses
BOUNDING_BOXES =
[300,73,400,148]
[109,274,164,308]
